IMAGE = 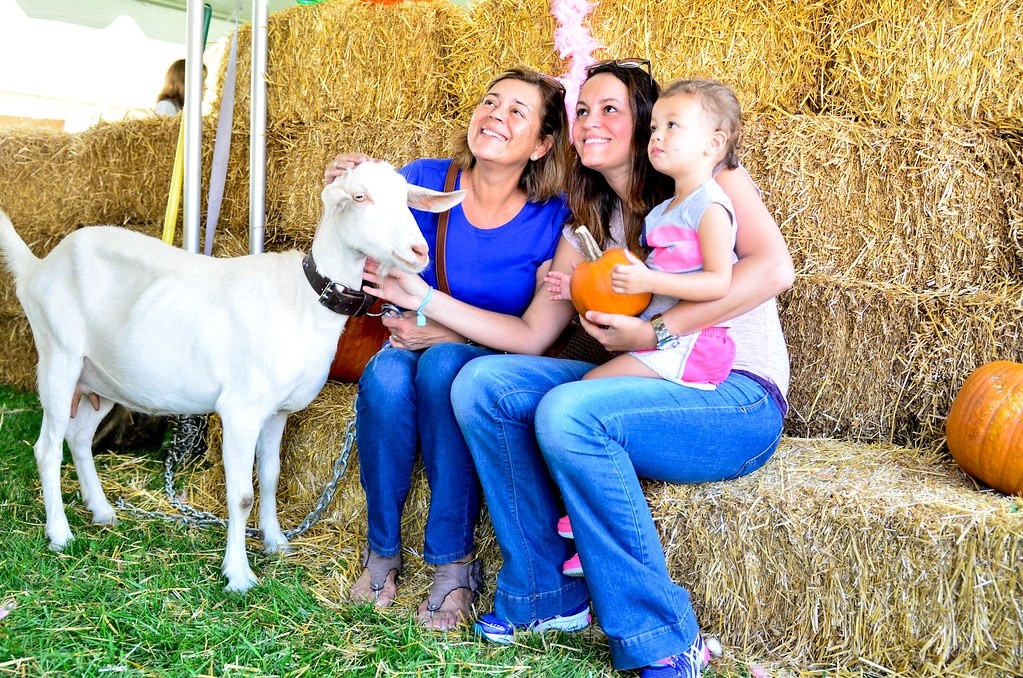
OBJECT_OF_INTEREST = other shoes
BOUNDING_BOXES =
[557,514,574,540]
[562,551,585,579]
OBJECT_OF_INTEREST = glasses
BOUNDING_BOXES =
[585,57,652,90]
[502,67,566,103]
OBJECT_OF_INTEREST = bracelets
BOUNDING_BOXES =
[416,284,434,327]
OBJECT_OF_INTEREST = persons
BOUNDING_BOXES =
[363,57,801,676]
[153,56,211,123]
[541,76,745,578]
[326,66,581,631]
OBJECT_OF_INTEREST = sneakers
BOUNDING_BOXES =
[473,596,591,646]
[642,632,710,678]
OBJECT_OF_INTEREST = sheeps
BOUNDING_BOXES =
[2,161,467,594]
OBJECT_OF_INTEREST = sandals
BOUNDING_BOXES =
[415,559,484,637]
[349,545,403,617]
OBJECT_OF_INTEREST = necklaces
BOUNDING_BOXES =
[634,245,647,261]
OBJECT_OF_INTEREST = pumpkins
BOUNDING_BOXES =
[328,298,393,380]
[946,357,1023,495]
[569,225,653,326]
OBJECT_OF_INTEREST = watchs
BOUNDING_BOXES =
[648,312,681,352]
